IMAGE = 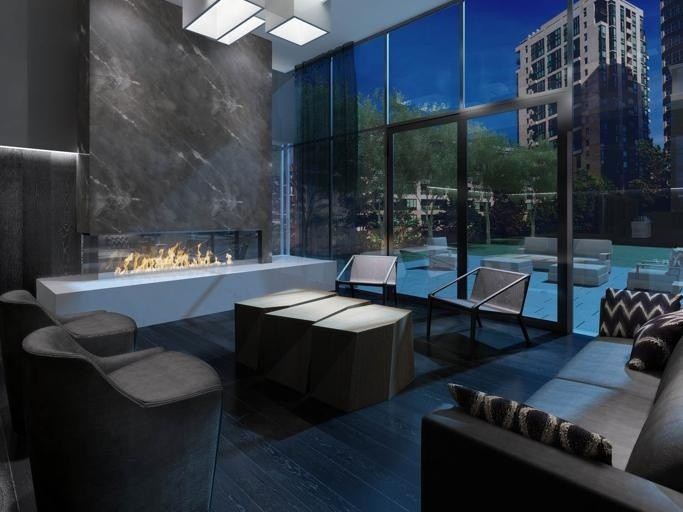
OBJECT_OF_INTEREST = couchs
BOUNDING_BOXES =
[481,236,612,287]
[399,236,456,269]
[626,246,682,290]
[413,284,682,512]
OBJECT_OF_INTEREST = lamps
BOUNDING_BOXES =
[180,0,331,47]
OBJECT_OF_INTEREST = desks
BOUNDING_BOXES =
[230,286,416,414]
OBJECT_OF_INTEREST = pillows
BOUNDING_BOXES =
[623,306,682,377]
[596,285,683,341]
[442,380,614,468]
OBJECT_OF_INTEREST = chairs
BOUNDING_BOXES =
[331,253,398,308]
[15,320,222,511]
[0,286,139,465]
[423,263,535,373]
